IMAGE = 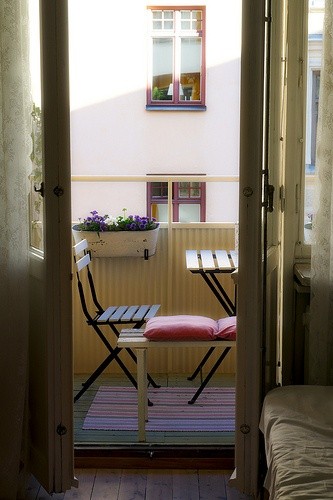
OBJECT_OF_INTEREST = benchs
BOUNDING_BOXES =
[116,315,236,441]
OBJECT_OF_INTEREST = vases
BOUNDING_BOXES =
[72,223,160,260]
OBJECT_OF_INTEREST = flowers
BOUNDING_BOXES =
[77,210,158,239]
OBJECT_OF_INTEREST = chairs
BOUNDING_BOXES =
[72,239,162,407]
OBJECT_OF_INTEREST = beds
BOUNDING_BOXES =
[260,382,333,499]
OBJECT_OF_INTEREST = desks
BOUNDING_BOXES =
[184,249,264,405]
